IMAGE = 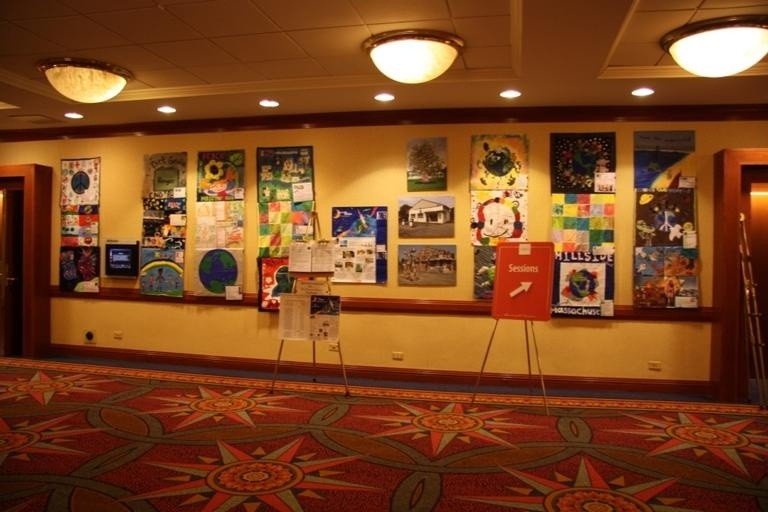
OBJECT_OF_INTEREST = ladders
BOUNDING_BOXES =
[739,212,768,409]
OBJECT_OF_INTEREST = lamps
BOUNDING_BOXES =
[37,58,132,104]
[660,15,768,78]
[361,30,464,84]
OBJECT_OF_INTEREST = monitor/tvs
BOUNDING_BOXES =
[100,239,140,280]
